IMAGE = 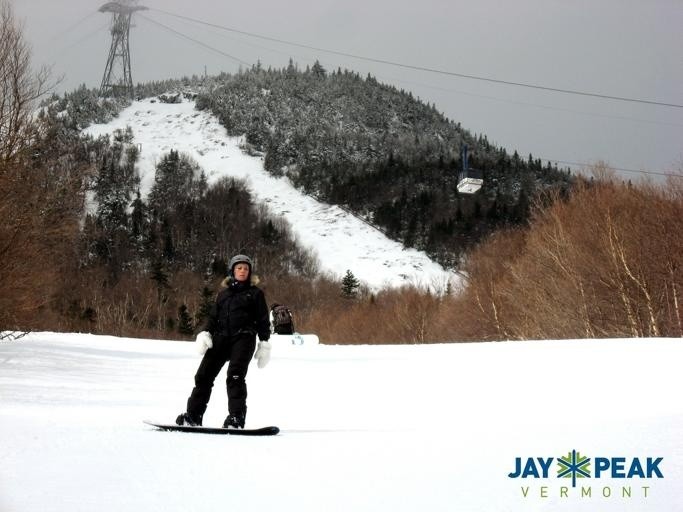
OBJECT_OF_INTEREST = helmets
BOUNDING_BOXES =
[229,254,253,269]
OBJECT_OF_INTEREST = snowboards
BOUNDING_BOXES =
[148,414,279,436]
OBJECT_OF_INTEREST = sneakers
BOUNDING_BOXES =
[176,413,202,426]
[223,414,245,428]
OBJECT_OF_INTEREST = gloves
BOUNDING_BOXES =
[196,331,213,355]
[254,341,271,368]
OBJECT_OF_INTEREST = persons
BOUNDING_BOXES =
[176,255,270,428]
[269,303,294,335]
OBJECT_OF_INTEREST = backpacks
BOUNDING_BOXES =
[273,306,295,335]
